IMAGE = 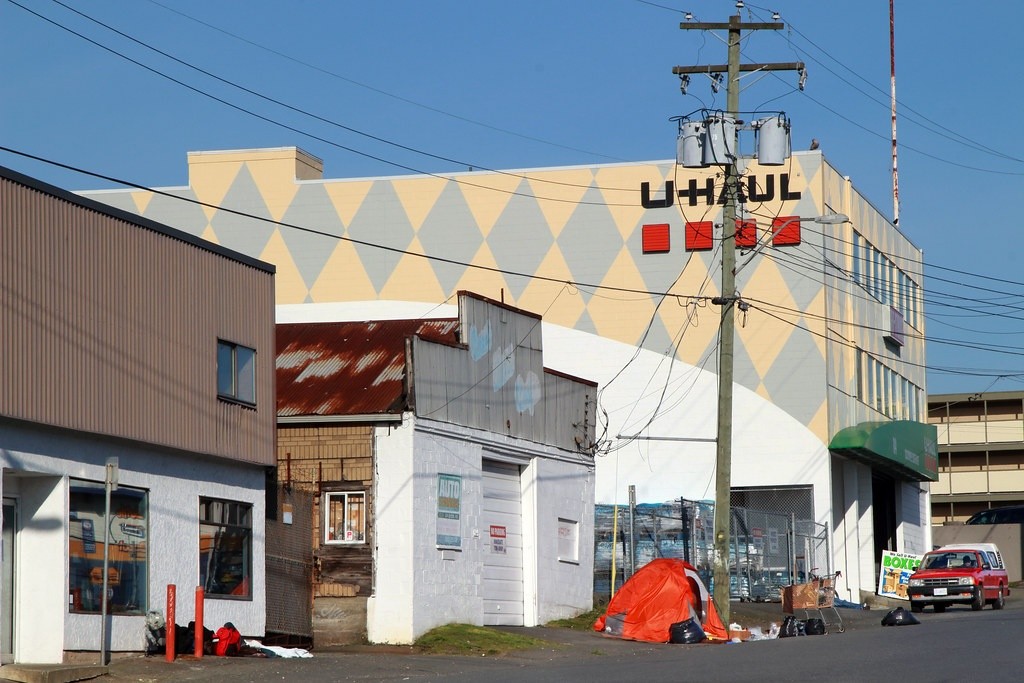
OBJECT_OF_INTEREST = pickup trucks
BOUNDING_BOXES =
[906,543,1011,613]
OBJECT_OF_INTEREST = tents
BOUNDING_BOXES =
[593,559,728,643]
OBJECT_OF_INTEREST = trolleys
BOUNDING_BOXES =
[780,574,845,635]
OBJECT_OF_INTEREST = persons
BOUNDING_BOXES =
[960,556,975,567]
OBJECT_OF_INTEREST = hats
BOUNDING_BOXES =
[963,555,971,563]
